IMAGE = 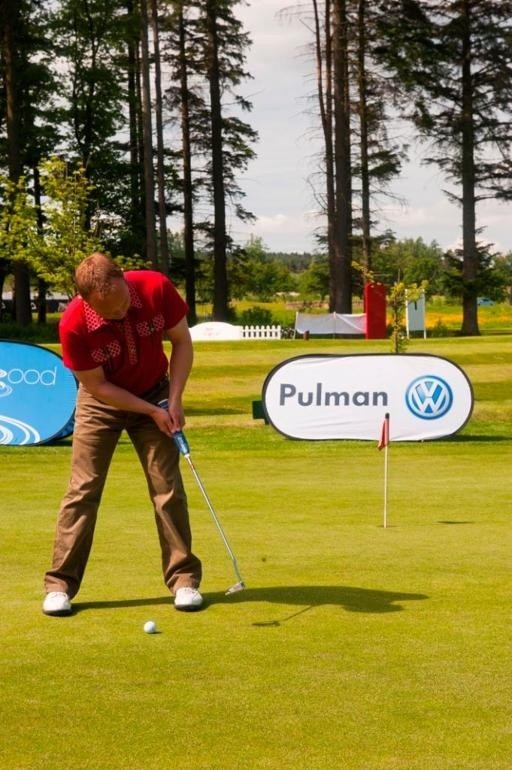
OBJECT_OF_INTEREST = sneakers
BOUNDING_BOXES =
[175,588,204,611]
[43,591,73,616]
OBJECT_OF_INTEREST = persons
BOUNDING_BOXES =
[40,250,205,615]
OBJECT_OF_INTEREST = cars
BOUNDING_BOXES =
[477,297,495,306]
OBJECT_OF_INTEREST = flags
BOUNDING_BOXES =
[377,421,388,452]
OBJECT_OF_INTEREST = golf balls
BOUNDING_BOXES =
[144,622,156,632]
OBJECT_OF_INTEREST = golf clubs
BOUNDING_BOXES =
[159,399,244,596]
